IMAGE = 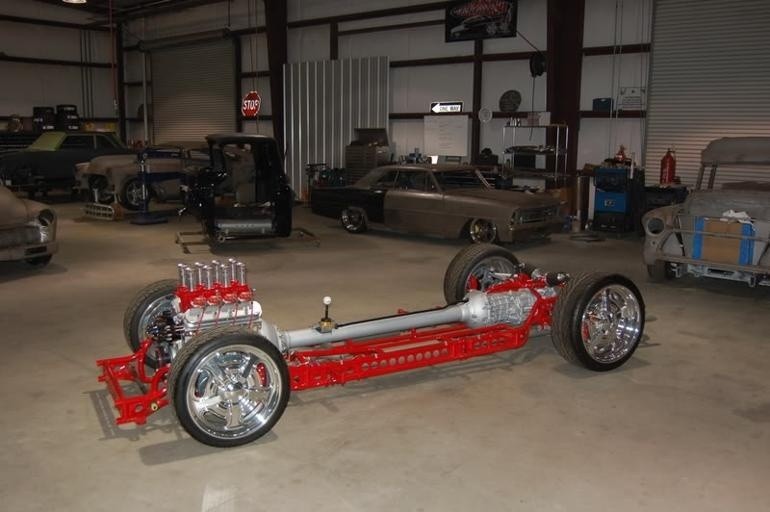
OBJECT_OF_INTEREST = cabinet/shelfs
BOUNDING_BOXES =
[499,124,569,190]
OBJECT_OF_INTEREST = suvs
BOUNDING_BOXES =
[638,134,770,295]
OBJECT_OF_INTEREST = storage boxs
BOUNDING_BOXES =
[691,220,755,264]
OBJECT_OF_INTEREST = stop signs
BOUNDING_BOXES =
[239,90,262,118]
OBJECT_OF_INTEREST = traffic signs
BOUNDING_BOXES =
[430,101,463,114]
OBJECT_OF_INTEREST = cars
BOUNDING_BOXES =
[451,15,501,40]
[0,128,138,191]
[75,139,253,211]
[0,177,59,271]
[306,158,563,251]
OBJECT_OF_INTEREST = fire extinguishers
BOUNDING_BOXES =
[660,148,676,186]
[614,144,627,161]
